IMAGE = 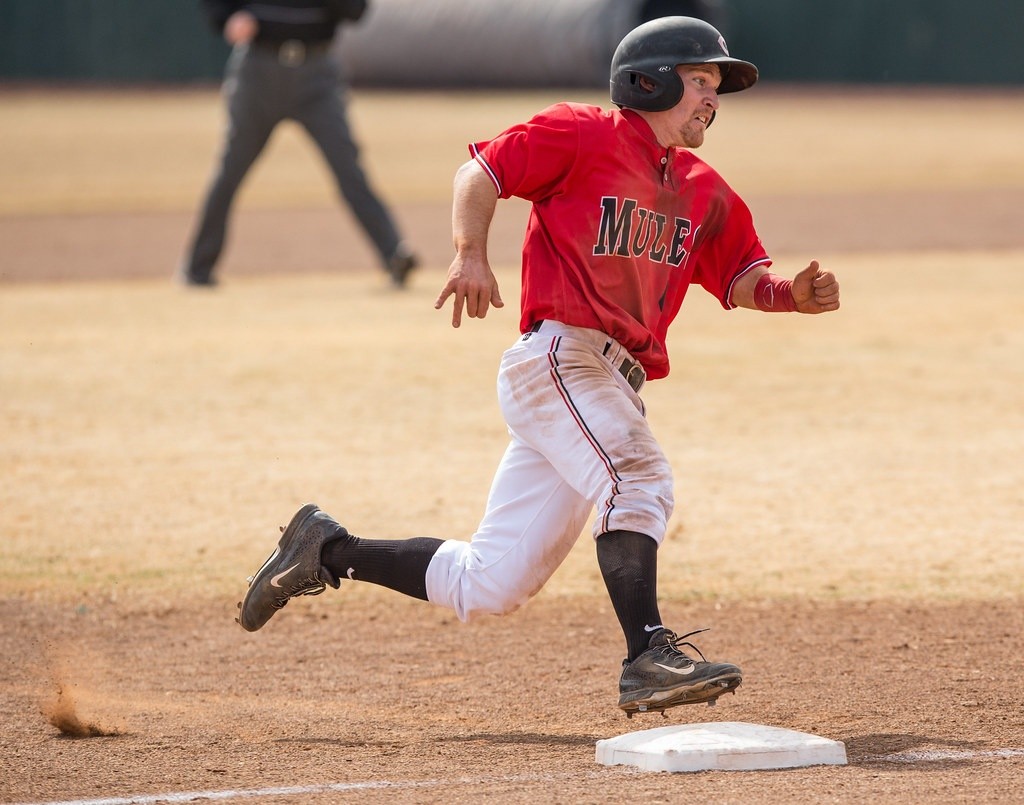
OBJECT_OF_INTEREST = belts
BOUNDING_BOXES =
[532,320,645,393]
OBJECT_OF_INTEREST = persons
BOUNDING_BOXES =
[185,0,415,287]
[237,15,839,720]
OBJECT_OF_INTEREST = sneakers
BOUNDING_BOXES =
[618,628,743,713]
[238,502,348,631]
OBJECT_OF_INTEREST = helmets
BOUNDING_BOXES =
[609,16,758,111]
